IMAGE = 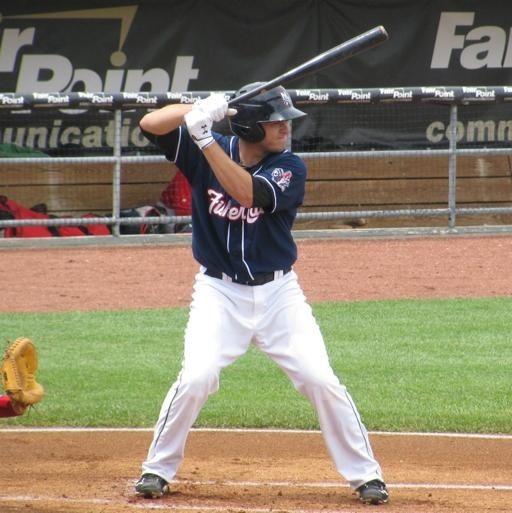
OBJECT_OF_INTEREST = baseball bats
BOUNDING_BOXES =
[226,25,389,107]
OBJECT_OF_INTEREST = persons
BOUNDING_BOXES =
[132,80,389,504]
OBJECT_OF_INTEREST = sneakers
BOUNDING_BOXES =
[135,474,169,499]
[358,480,389,503]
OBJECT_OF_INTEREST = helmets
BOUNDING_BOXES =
[227,82,306,142]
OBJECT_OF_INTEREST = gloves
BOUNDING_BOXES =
[186,110,214,149]
[195,95,237,121]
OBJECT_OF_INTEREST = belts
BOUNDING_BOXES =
[204,267,290,287]
